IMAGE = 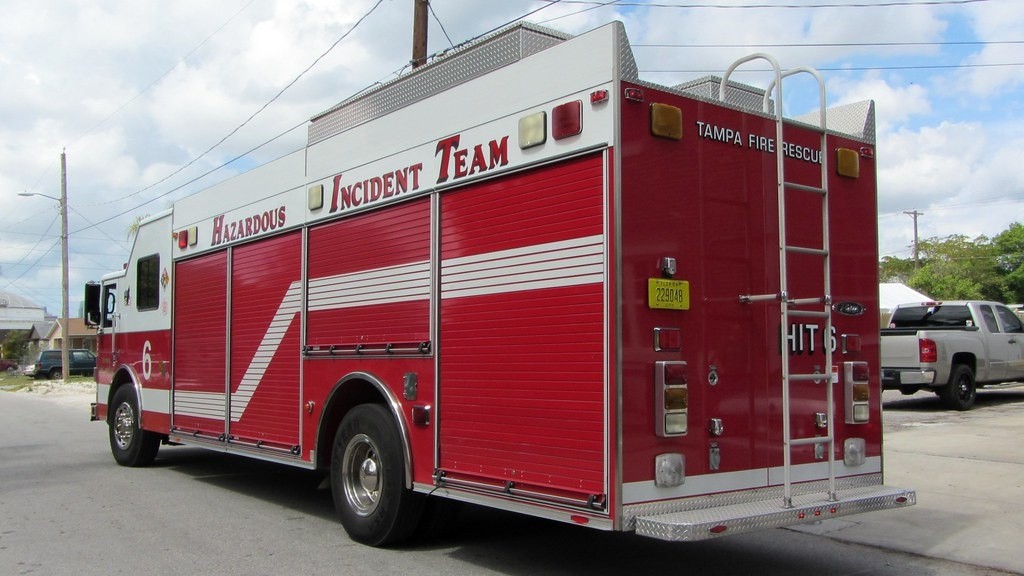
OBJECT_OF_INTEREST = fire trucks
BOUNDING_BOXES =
[83,24,917,554]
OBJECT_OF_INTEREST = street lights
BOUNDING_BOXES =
[18,190,70,381]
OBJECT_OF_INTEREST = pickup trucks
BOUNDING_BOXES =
[880,300,1024,409]
[0,358,18,374]
[23,349,97,381]
